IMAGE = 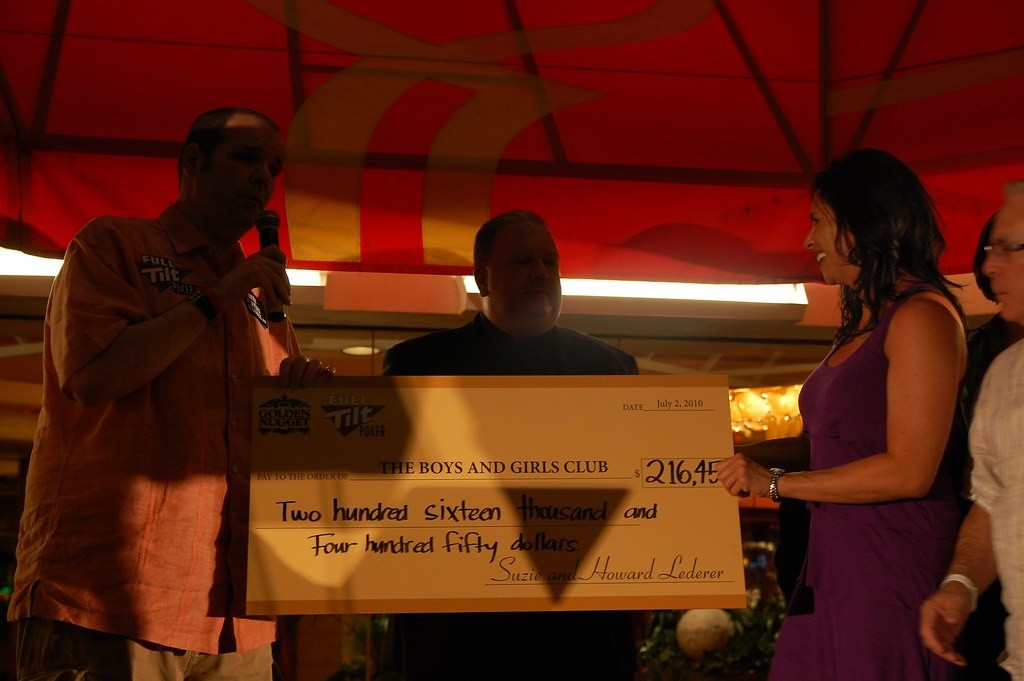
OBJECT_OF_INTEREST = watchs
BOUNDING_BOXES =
[768,466,786,503]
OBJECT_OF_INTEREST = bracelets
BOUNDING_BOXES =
[940,574,978,613]
[187,289,220,324]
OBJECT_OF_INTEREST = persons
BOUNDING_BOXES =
[5,107,337,681]
[714,147,971,681]
[378,209,655,681]
[920,179,1024,681]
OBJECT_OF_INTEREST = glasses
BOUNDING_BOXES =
[983,243,1024,256]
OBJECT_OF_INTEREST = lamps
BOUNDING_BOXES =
[455,275,809,322]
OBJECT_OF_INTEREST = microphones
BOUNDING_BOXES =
[255,209,288,325]
